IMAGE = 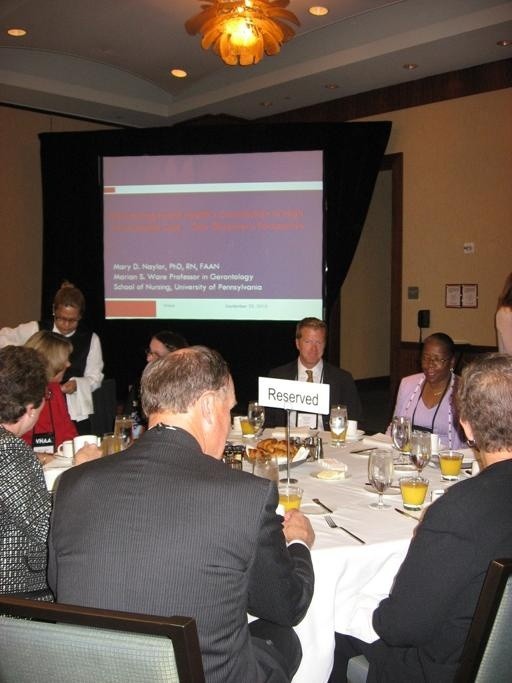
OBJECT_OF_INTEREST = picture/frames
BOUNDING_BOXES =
[445,284,478,308]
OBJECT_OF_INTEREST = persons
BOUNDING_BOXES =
[19,330,79,467]
[326,352,512,682]
[115,330,188,439]
[0,346,104,623]
[0,282,104,435]
[46,346,316,683]
[261,317,362,432]
[493,270,512,359]
[385,332,470,456]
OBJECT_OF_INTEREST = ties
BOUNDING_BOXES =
[304,369,314,382]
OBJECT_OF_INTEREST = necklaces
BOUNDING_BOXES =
[427,383,447,397]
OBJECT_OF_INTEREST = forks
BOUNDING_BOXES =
[324,515,365,544]
[312,498,332,513]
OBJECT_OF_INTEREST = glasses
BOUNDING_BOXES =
[51,304,83,325]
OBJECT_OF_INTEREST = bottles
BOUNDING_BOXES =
[290,435,324,462]
[224,446,242,463]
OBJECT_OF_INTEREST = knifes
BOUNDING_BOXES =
[394,507,421,523]
[351,447,378,453]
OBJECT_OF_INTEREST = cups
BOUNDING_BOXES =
[58,416,135,458]
[250,457,304,514]
[234,402,265,437]
[228,461,243,470]
[329,404,365,446]
[400,433,481,511]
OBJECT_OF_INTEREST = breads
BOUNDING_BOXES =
[249,439,298,461]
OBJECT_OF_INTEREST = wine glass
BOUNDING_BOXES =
[367,416,431,510]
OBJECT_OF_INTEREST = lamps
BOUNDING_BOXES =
[184,0,301,67]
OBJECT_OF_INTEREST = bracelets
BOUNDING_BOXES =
[43,452,47,466]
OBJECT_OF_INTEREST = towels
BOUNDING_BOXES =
[271,427,311,438]
[363,432,402,451]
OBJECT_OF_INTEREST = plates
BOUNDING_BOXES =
[299,502,334,516]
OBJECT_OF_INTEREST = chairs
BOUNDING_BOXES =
[0,594,205,683]
[346,559,512,683]
